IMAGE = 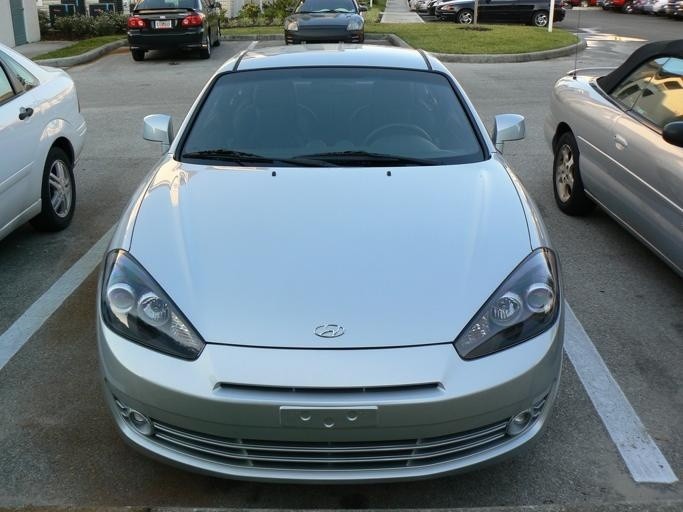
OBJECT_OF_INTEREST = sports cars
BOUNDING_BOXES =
[543,37,683,283]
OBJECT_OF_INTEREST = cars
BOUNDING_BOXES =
[125,0,224,62]
[281,0,367,47]
[91,33,569,488]
[0,40,89,246]
[405,0,682,27]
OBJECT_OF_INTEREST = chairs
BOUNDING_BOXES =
[229,72,436,155]
